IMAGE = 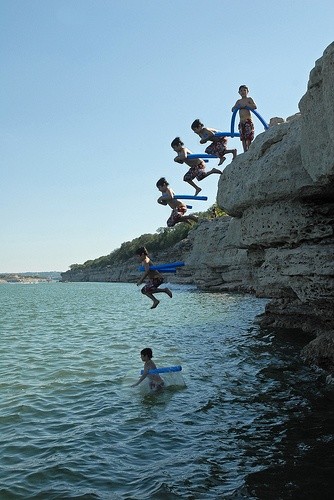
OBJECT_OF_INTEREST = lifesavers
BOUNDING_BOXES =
[204,131,240,138]
[141,366,182,375]
[174,153,219,163]
[136,261,185,274]
[157,194,207,209]
[231,104,270,138]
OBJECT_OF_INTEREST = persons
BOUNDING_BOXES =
[188,119,245,166]
[231,85,270,152]
[135,244,185,310]
[171,137,222,199]
[129,348,185,393]
[155,177,207,227]
